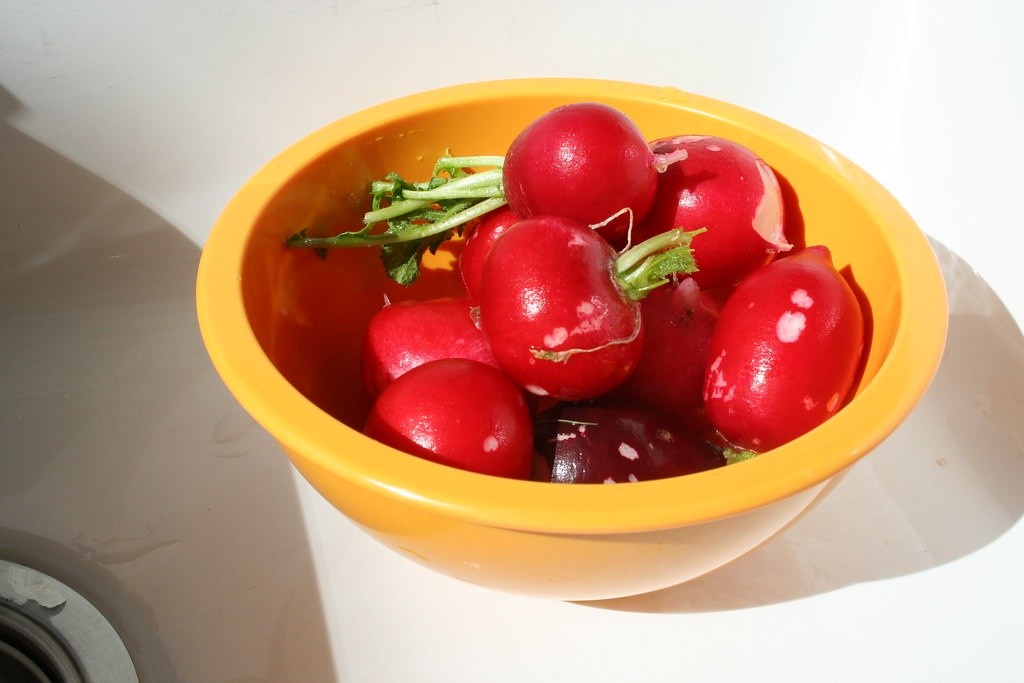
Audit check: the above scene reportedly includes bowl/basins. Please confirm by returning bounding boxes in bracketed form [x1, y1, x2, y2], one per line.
[196, 77, 949, 602]
